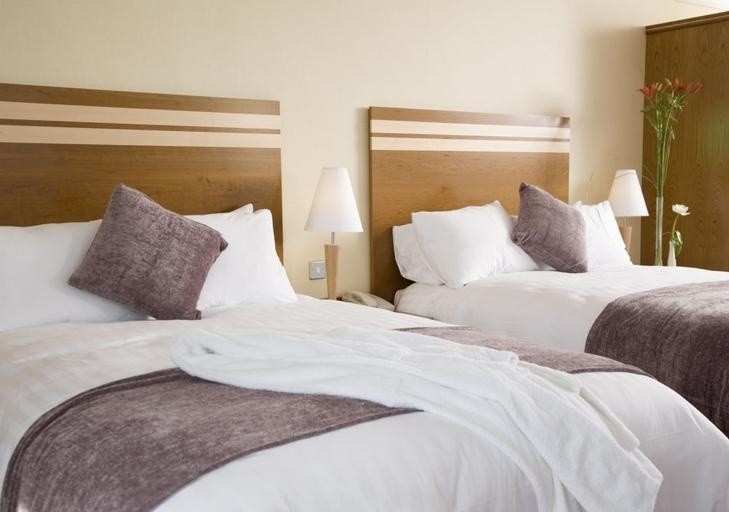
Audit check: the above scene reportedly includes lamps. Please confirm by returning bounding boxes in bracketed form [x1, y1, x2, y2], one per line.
[304, 167, 363, 300]
[608, 170, 650, 253]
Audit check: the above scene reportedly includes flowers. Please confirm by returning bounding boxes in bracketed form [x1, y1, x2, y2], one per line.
[661, 204, 692, 254]
[638, 77, 701, 264]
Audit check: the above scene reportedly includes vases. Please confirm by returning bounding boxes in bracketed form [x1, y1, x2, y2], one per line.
[667, 240, 678, 266]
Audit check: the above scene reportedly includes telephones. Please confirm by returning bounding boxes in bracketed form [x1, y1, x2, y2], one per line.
[342, 290, 395, 312]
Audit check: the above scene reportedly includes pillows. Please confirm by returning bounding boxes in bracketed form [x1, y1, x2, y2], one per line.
[391, 182, 632, 287]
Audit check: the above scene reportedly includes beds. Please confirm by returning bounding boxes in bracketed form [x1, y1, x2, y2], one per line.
[0, 83, 729, 511]
[370, 106, 728, 439]
